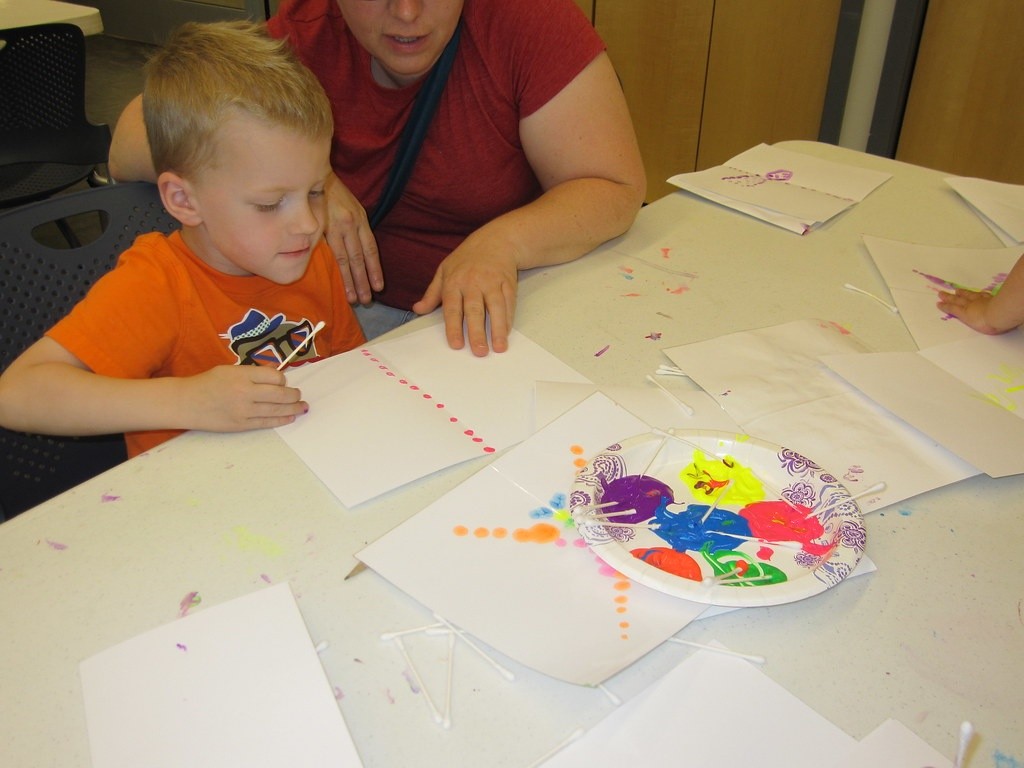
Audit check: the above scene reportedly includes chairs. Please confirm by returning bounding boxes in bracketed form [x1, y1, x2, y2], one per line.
[0, 22, 115, 249]
[0, 180, 183, 526]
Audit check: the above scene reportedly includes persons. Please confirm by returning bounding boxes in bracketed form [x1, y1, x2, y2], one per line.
[104, 1, 649, 355]
[936, 253, 1023, 334]
[1, 16, 368, 461]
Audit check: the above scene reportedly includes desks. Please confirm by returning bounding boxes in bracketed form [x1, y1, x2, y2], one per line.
[0, 0, 104, 50]
[0, 138, 1023, 765]
